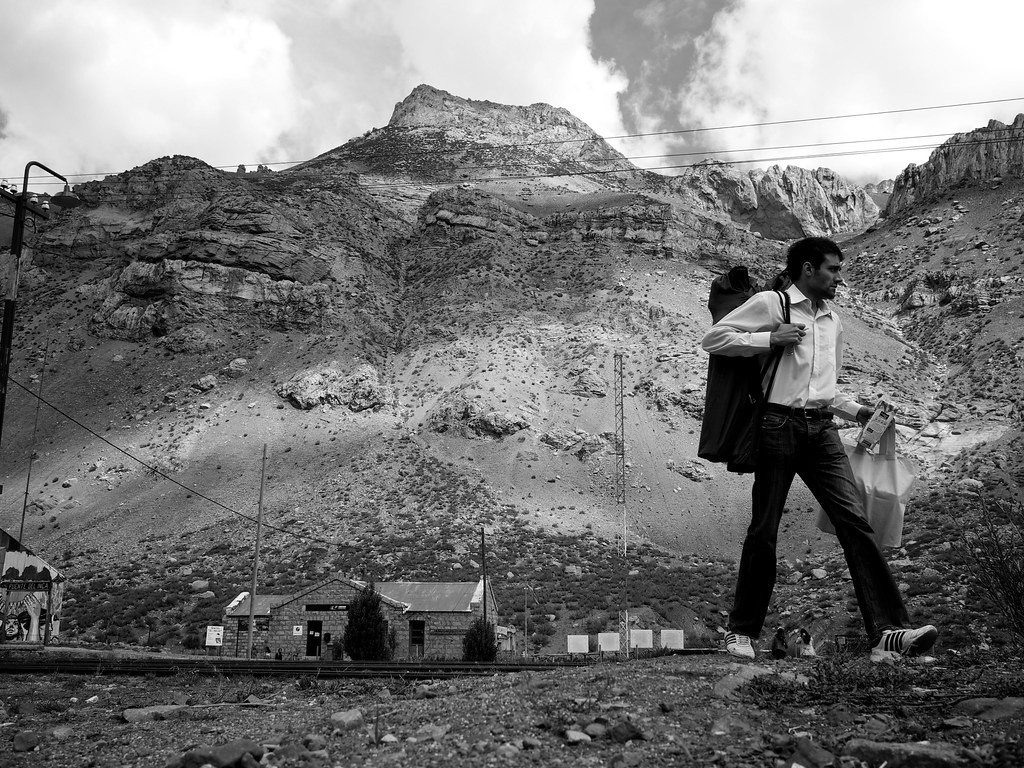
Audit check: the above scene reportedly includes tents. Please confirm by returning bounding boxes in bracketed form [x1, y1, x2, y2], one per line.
[0, 528, 66, 644]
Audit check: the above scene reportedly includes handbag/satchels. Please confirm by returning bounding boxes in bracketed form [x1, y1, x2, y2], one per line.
[814, 415, 919, 548]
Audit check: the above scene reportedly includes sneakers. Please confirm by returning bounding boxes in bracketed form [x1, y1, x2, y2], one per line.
[869, 624, 938, 663]
[724, 631, 757, 661]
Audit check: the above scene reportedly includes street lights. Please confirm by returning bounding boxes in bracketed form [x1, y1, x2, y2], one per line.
[0, 160, 82, 443]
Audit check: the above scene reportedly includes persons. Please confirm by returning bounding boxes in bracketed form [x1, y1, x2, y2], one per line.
[264, 647, 271, 658]
[702, 240, 938, 658]
[275, 648, 282, 660]
